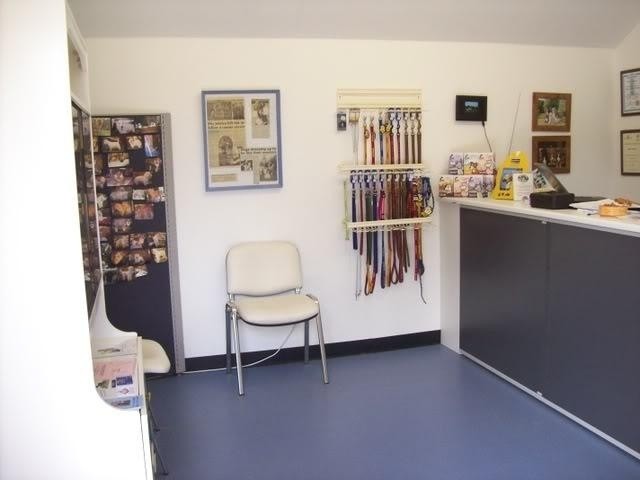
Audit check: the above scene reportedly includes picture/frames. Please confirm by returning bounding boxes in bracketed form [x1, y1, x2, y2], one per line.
[201, 88, 285, 191]
[619, 68, 640, 116]
[533, 136, 571, 173]
[619, 128, 640, 176]
[532, 92, 572, 131]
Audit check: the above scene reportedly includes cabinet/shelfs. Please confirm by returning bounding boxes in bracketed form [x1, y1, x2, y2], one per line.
[337, 88, 434, 241]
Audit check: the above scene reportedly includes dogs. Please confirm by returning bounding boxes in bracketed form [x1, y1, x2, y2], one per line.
[104, 139, 122, 152]
[133, 172, 152, 186]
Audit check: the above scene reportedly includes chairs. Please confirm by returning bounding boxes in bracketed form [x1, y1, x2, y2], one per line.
[223, 240, 330, 396]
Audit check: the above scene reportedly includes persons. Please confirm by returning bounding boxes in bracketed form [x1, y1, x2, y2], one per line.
[208, 99, 277, 180]
[538, 106, 563, 168]
